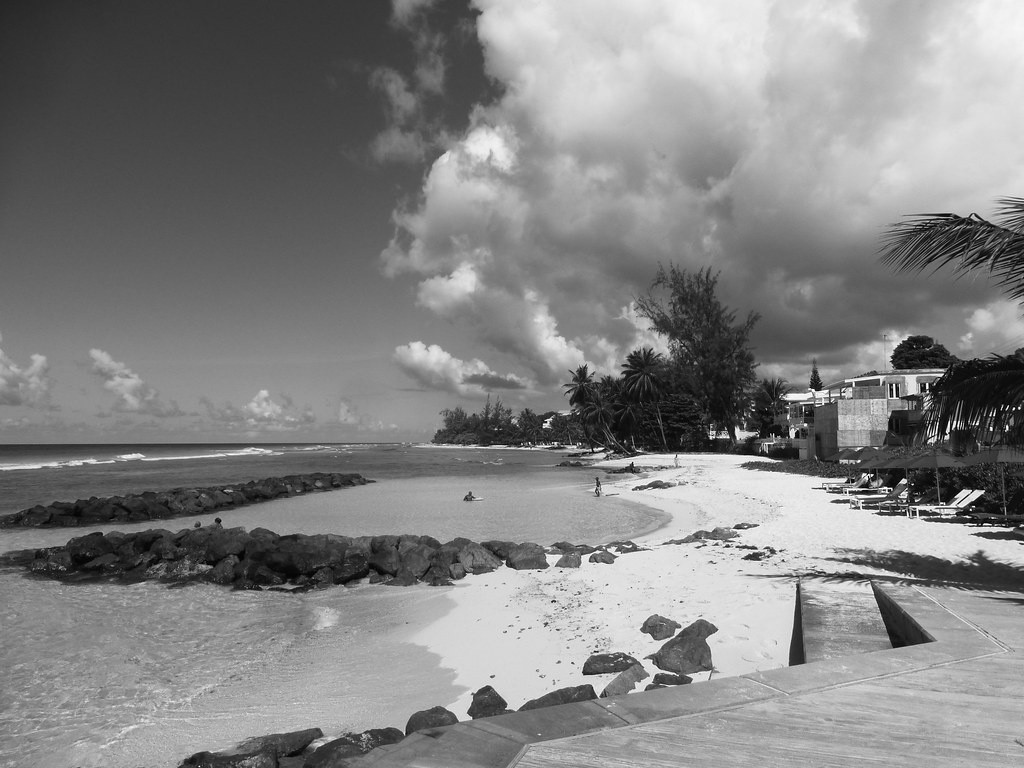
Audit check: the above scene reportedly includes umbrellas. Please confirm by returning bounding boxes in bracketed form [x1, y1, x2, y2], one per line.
[824, 445, 1024, 516]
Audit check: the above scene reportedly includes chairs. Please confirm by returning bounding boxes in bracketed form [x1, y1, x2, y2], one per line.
[819, 473, 985, 526]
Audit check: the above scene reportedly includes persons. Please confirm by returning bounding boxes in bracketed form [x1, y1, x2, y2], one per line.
[594, 477, 601, 496]
[463, 491, 475, 501]
